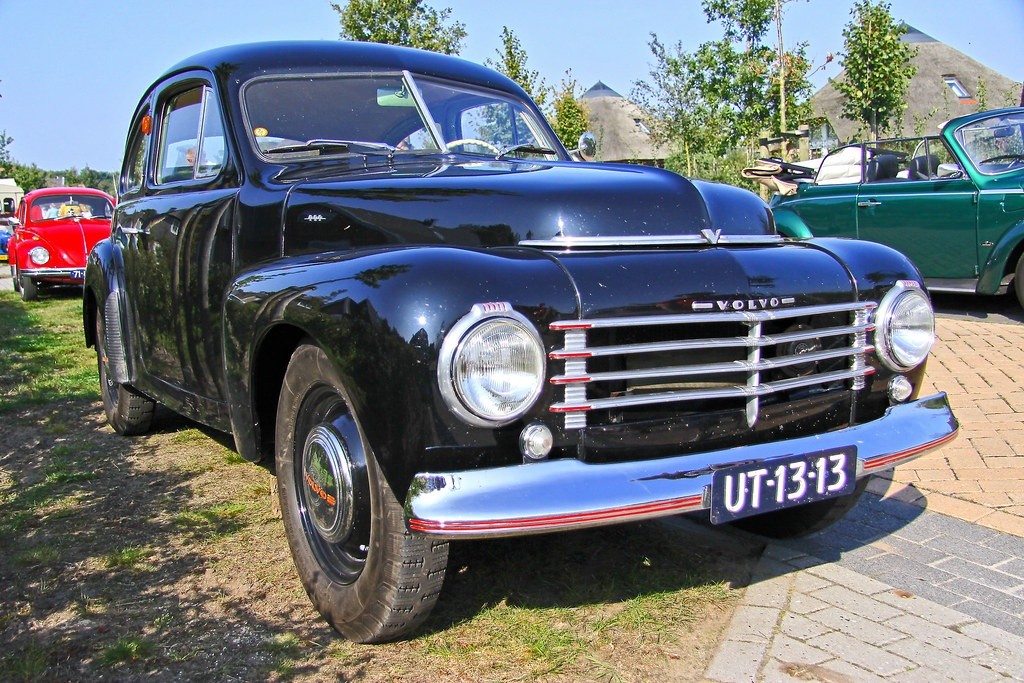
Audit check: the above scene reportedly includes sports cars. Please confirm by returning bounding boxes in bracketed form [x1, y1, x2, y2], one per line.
[768, 104, 1024, 313]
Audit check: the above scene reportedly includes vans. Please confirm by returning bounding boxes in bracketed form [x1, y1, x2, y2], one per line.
[0, 178, 27, 261]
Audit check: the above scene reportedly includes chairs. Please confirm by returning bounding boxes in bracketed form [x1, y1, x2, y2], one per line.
[908, 155, 940, 181]
[866, 155, 910, 184]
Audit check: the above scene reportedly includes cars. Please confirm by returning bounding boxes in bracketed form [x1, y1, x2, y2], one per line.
[82, 39, 961, 644]
[4, 186, 117, 302]
